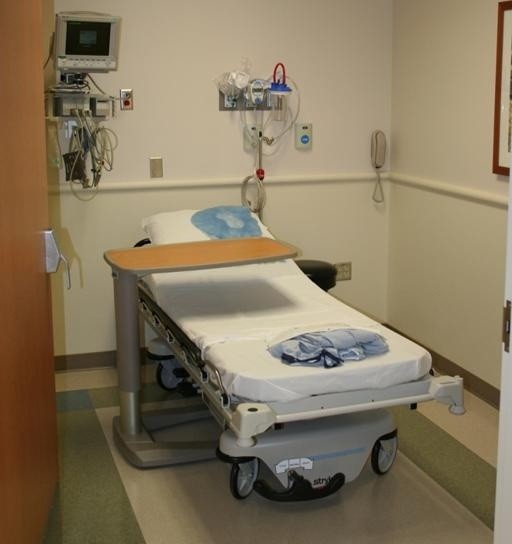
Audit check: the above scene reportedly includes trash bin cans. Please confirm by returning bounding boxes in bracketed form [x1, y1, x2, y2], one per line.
[295, 260, 339, 292]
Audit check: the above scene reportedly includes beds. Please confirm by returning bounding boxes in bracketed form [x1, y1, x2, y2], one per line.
[105, 206, 467, 508]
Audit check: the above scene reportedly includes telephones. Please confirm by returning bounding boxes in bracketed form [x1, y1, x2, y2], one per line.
[371, 131, 386, 168]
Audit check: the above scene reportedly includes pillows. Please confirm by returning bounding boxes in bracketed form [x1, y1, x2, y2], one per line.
[143, 207, 298, 282]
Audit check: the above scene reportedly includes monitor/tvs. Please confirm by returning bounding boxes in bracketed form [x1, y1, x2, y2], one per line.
[48, 10, 120, 74]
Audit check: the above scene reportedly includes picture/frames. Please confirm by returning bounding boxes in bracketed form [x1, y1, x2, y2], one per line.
[493, 1, 509, 175]
